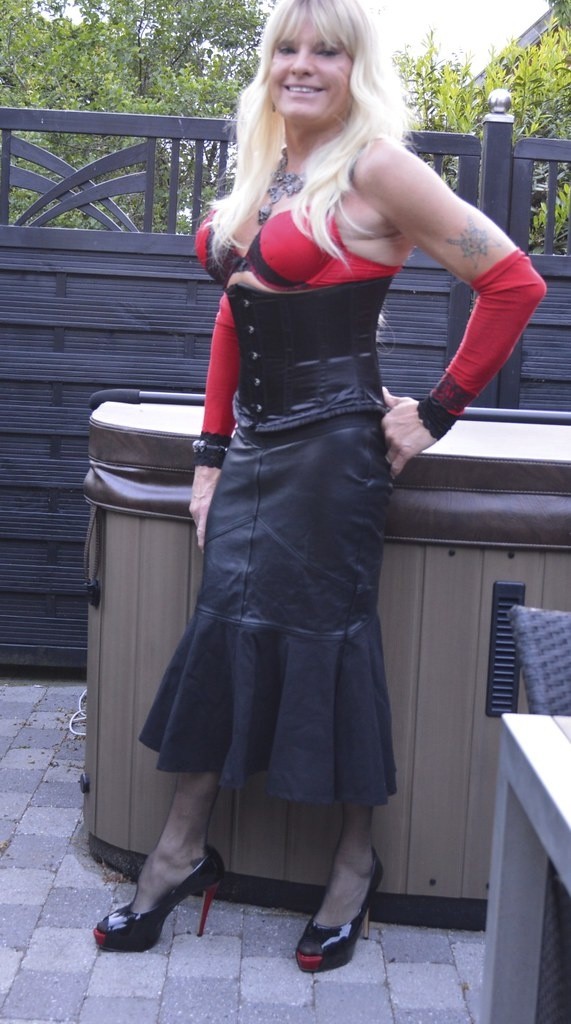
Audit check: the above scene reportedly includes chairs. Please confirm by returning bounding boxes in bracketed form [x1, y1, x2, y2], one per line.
[510, 604, 570, 1024]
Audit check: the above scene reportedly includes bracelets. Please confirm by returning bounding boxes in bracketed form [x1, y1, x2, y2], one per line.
[192, 440, 228, 453]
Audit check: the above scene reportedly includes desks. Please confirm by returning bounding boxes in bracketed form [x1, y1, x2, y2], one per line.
[481, 712, 570, 1024]
[80, 388, 570, 931]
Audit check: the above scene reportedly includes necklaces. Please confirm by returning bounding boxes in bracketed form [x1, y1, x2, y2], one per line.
[259, 146, 305, 226]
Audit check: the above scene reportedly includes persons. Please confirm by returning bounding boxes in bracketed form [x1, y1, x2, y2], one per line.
[93, 0, 547, 972]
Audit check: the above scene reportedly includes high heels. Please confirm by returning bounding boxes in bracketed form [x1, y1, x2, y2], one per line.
[295, 845, 383, 972]
[93, 844, 225, 953]
